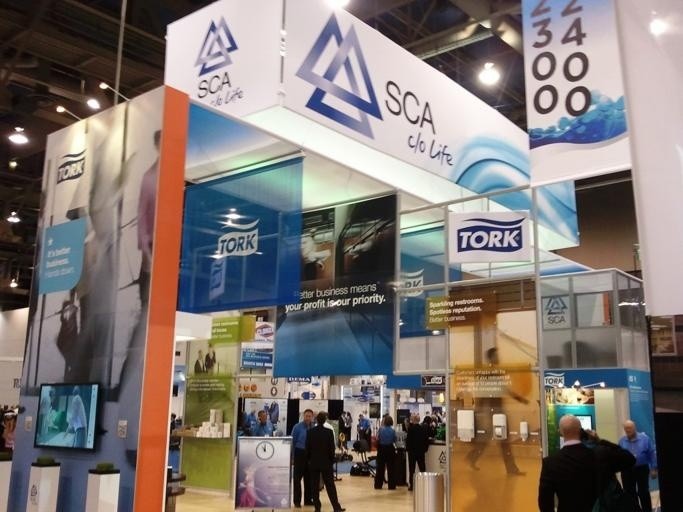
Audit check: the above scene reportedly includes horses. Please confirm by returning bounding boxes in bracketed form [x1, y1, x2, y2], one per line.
[580, 429, 590, 441]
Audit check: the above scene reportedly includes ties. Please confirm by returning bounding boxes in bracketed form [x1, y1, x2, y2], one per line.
[576, 415, 592, 430]
[34, 382, 100, 451]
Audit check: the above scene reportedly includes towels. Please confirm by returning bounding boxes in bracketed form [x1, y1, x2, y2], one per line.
[412, 471, 444, 512]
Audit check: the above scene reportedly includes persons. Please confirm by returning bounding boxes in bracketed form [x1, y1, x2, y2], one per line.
[252, 410, 274, 436]
[60, 384, 88, 448]
[462, 346, 528, 476]
[618, 419, 658, 512]
[538, 413, 636, 512]
[194, 344, 216, 373]
[137, 131, 160, 308]
[36, 385, 56, 437]
[291, 409, 446, 512]
